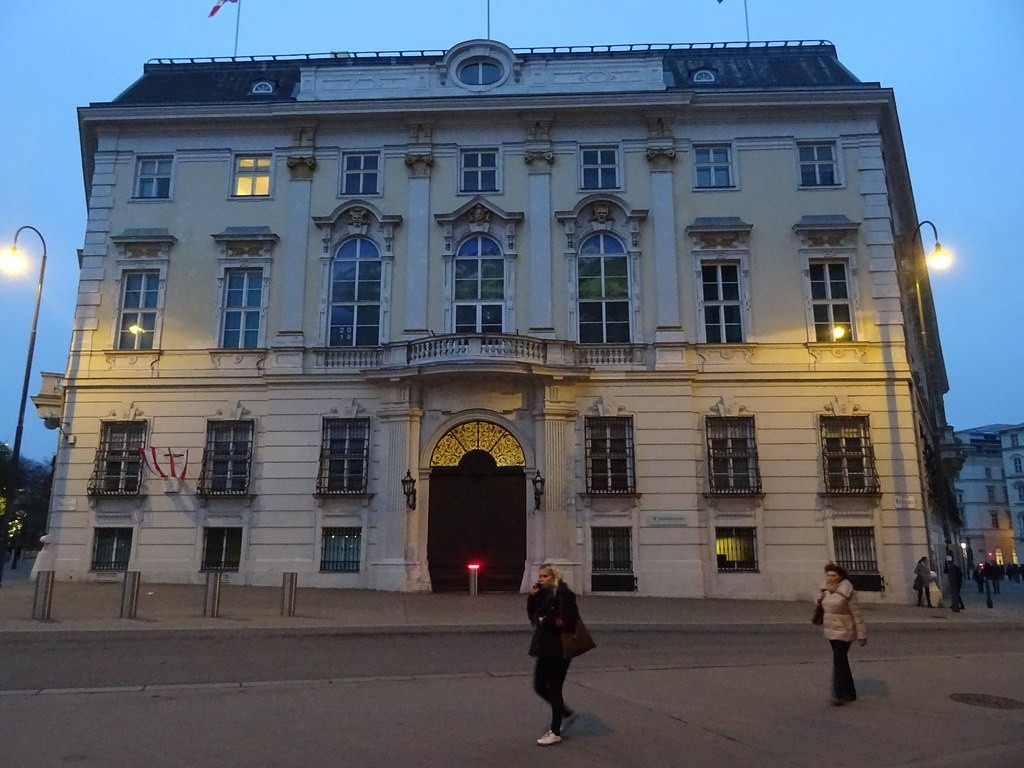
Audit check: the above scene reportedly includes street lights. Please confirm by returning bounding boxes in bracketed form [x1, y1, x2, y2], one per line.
[911, 220, 962, 611]
[1, 224, 49, 575]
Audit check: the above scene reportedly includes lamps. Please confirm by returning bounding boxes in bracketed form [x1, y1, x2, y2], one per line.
[533, 470, 545, 511]
[467, 565, 480, 595]
[401, 469, 416, 510]
[44, 416, 70, 440]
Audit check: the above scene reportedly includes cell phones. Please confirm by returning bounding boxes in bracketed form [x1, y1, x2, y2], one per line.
[534, 583, 540, 590]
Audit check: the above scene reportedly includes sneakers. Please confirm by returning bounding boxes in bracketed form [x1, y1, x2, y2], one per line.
[537, 729, 562, 745]
[560, 708, 579, 732]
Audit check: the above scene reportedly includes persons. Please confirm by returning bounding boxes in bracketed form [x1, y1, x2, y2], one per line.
[526, 563, 580, 745]
[914, 556, 936, 608]
[943, 556, 965, 613]
[972, 562, 1024, 594]
[820, 563, 868, 706]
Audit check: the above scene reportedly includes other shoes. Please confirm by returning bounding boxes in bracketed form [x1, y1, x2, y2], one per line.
[829, 695, 850, 706]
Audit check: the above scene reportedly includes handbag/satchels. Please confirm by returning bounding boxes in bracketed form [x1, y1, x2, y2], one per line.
[812, 604, 824, 625]
[559, 594, 596, 659]
[913, 574, 922, 591]
